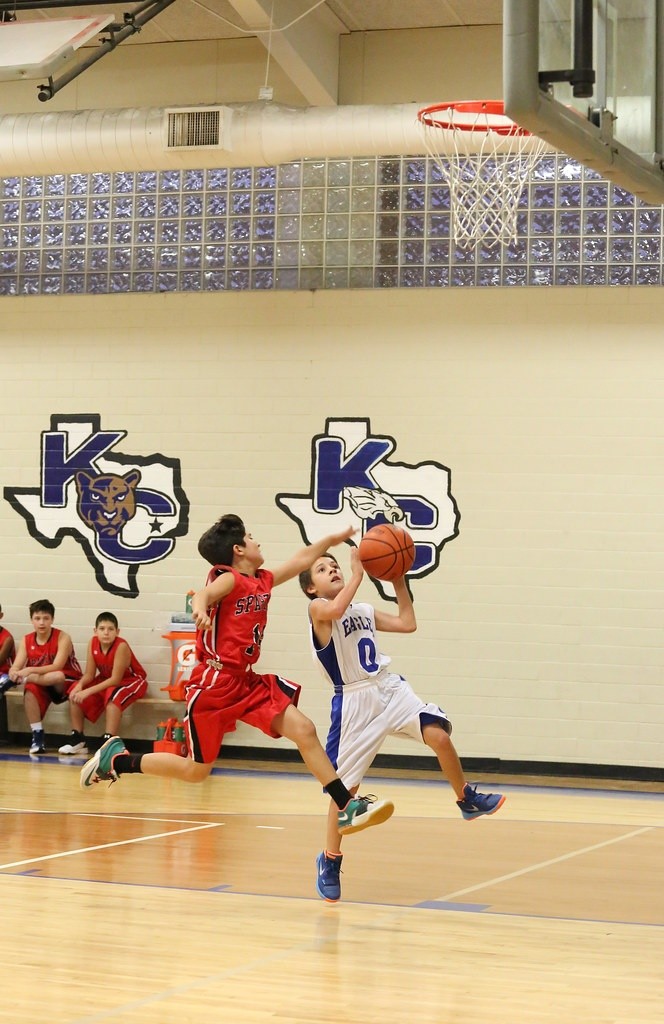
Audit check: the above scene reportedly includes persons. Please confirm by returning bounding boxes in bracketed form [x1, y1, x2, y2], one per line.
[79, 513, 394, 833]
[299, 547, 505, 902]
[8, 599, 82, 753]
[58, 612, 148, 754]
[0, 606, 16, 746]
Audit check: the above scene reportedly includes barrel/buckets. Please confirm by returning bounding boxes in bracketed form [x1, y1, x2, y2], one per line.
[160, 623, 198, 702]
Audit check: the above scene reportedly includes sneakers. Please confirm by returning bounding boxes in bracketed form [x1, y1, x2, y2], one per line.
[337, 793, 395, 835]
[456, 782, 506, 821]
[316, 851, 344, 902]
[30, 727, 47, 754]
[0, 674, 22, 700]
[58, 729, 89, 755]
[97, 733, 112, 750]
[80, 735, 130, 791]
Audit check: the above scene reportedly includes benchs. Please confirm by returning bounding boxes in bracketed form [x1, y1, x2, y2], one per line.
[3, 686, 191, 703]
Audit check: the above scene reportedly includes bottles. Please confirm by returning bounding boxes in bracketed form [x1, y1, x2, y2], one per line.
[186, 590, 195, 612]
[182, 723, 186, 741]
[167, 718, 177, 740]
[172, 722, 183, 741]
[157, 721, 167, 740]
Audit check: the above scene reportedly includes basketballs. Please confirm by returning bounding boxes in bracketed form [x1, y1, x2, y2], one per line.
[360, 523, 417, 581]
